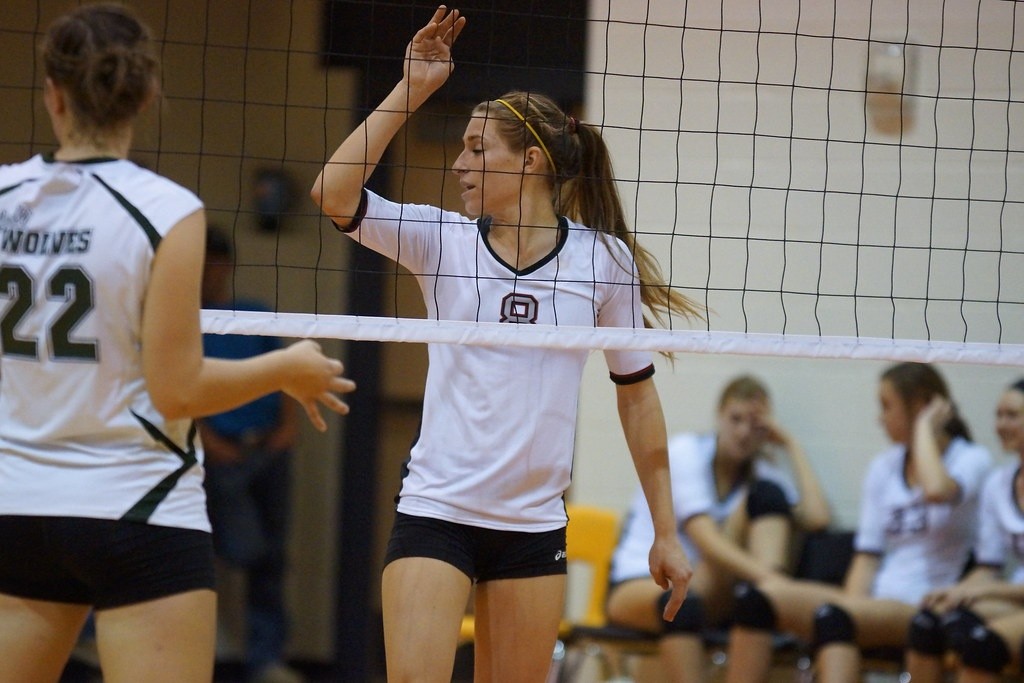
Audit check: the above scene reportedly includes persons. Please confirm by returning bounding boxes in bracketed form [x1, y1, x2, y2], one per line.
[603, 362, 1024, 683]
[0, 2, 356, 683]
[308, 4, 694, 683]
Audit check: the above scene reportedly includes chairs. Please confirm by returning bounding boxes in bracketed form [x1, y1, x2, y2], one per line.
[454, 503, 856, 683]
